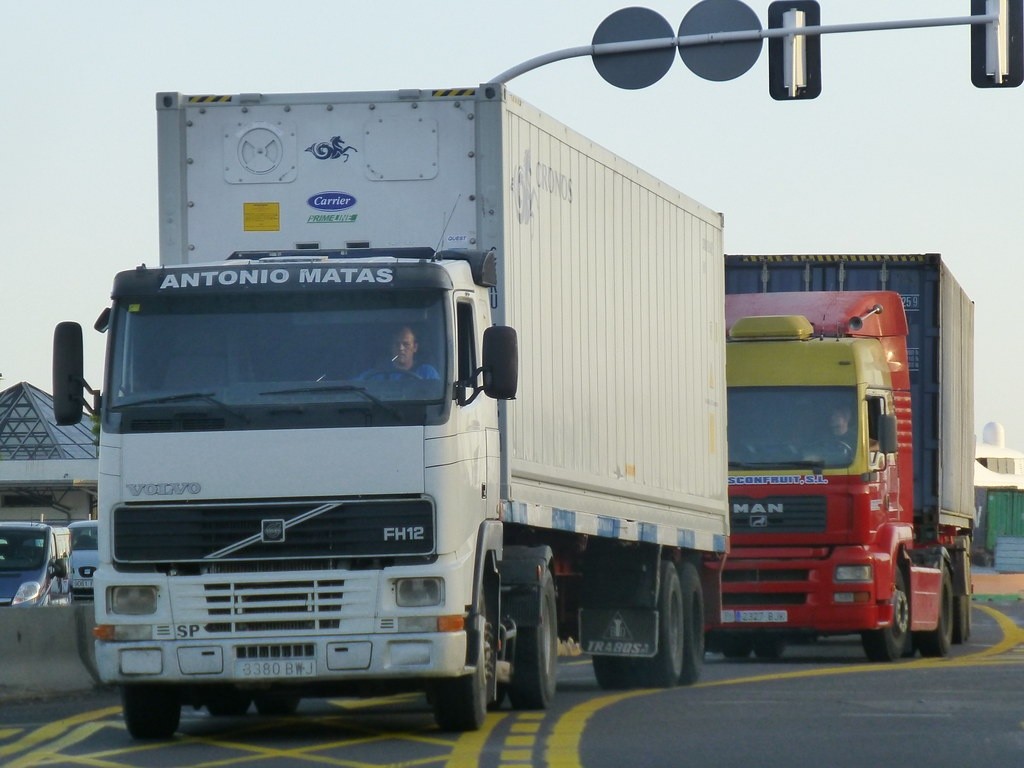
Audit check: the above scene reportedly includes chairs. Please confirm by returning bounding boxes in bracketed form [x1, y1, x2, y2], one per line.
[161, 325, 255, 390]
[77, 535, 93, 548]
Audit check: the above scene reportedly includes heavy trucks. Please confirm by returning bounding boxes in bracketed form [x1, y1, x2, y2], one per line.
[700, 251, 981, 662]
[49, 82, 728, 739]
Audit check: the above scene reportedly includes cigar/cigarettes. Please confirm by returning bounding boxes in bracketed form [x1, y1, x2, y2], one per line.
[391, 355, 398, 362]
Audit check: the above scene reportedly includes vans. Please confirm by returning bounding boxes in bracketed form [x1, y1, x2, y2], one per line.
[0, 519, 74, 608]
[65, 518, 98, 594]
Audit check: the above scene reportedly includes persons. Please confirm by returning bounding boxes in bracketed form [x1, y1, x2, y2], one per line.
[804, 409, 879, 454]
[358, 323, 440, 382]
